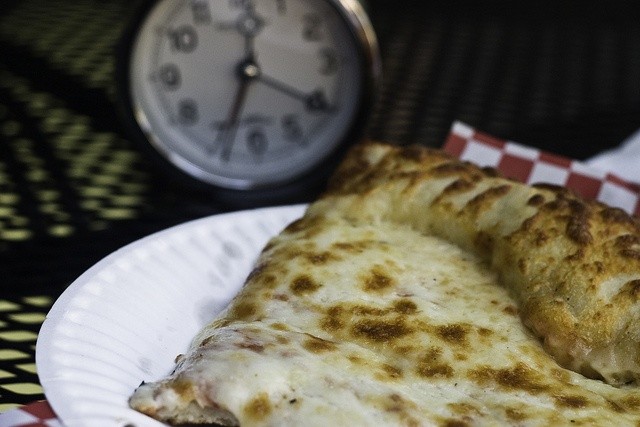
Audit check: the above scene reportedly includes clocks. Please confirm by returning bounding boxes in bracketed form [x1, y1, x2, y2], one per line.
[116, 1, 382, 207]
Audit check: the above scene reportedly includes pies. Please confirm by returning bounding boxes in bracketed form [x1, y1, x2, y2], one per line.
[127, 141, 640, 427]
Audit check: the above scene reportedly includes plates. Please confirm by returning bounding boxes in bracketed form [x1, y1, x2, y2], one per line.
[35, 203, 312, 427]
